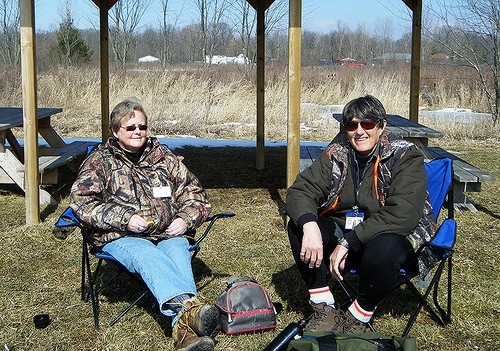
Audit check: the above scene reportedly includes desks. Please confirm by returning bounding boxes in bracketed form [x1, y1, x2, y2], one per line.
[333, 114, 443, 159]
[0, 107, 67, 205]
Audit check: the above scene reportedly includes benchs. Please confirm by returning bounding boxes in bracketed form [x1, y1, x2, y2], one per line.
[298, 146, 323, 173]
[19, 141, 91, 185]
[428, 147, 496, 192]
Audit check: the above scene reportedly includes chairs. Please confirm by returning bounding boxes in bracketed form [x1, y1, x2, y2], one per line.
[324, 157, 458, 338]
[54, 144, 236, 329]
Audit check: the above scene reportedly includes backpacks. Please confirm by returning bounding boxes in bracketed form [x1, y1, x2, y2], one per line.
[214, 275, 278, 335]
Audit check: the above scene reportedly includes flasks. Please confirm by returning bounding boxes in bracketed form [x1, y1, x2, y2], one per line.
[263, 317, 310, 351]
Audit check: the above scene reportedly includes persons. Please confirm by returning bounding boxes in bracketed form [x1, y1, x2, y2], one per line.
[70, 100, 221, 351]
[284, 94, 435, 333]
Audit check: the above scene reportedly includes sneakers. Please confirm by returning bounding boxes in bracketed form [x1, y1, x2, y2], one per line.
[171, 319, 215, 351]
[332, 310, 367, 336]
[178, 296, 220, 337]
[303, 299, 340, 337]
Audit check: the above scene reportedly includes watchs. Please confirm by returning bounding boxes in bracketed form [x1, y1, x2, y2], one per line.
[337, 237, 350, 249]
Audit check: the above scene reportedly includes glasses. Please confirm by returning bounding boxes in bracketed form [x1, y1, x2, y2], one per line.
[344, 120, 380, 131]
[120, 125, 148, 131]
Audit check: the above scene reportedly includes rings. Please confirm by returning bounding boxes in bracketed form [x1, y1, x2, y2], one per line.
[137, 225, 141, 231]
